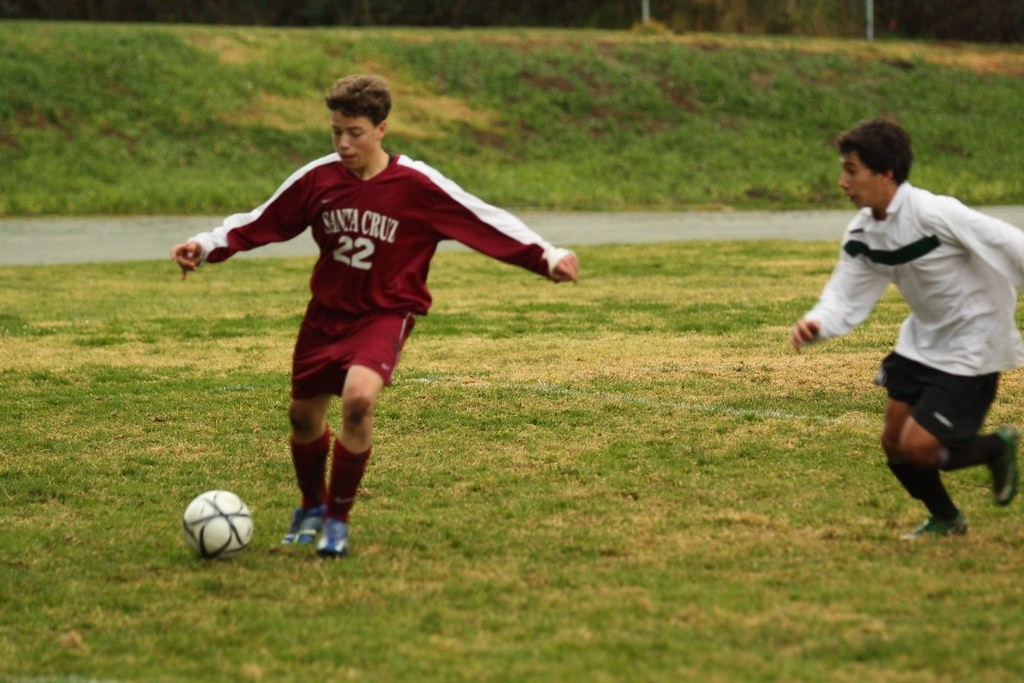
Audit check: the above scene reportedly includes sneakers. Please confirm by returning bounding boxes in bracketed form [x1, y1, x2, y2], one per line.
[994, 425, 1020, 505]
[318, 519, 348, 555]
[282, 505, 326, 547]
[900, 510, 968, 543]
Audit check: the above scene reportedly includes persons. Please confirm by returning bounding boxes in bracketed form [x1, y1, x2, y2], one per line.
[787, 114, 1024, 535]
[169, 72, 579, 560]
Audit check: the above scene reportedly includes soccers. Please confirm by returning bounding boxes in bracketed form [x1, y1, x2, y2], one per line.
[181, 488, 254, 562]
